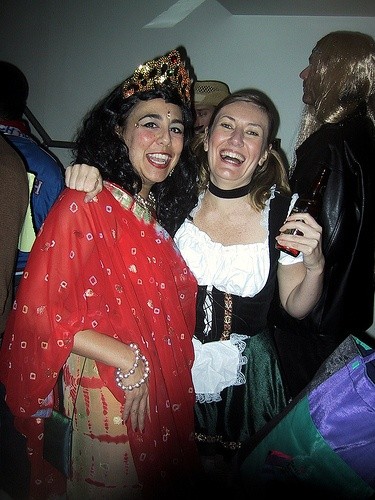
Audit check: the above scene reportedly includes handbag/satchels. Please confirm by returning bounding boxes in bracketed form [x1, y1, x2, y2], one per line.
[237, 334, 375, 500]
[43, 409, 73, 478]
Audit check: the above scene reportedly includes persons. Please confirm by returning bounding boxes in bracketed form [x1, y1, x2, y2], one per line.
[1, 64, 64, 300]
[191, 82, 230, 133]
[289, 32, 375, 362]
[65, 89, 325, 493]
[1, 75, 198, 496]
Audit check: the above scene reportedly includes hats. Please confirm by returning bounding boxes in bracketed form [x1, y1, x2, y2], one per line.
[192, 81, 231, 107]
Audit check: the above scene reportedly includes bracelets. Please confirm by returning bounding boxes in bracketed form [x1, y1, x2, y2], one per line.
[115, 344, 151, 392]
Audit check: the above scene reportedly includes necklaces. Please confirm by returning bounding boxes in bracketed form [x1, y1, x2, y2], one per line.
[134, 191, 157, 211]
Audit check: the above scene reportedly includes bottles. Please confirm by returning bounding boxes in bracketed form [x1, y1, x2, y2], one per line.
[275, 168, 332, 256]
